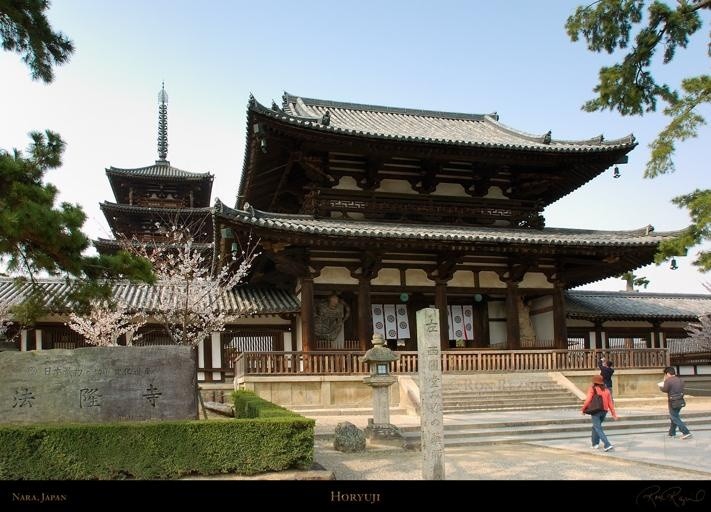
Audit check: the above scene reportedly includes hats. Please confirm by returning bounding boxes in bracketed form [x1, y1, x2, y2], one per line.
[591, 375, 604, 385]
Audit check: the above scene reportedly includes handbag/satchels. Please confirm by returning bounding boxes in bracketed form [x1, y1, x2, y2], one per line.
[668, 394, 686, 410]
[583, 394, 604, 415]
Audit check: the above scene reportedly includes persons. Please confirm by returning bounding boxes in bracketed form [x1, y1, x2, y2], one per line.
[582, 356, 618, 453]
[657, 366, 694, 439]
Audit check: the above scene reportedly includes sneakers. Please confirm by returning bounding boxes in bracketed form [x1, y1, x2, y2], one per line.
[604, 445, 613, 452]
[681, 433, 694, 439]
[590, 444, 600, 450]
